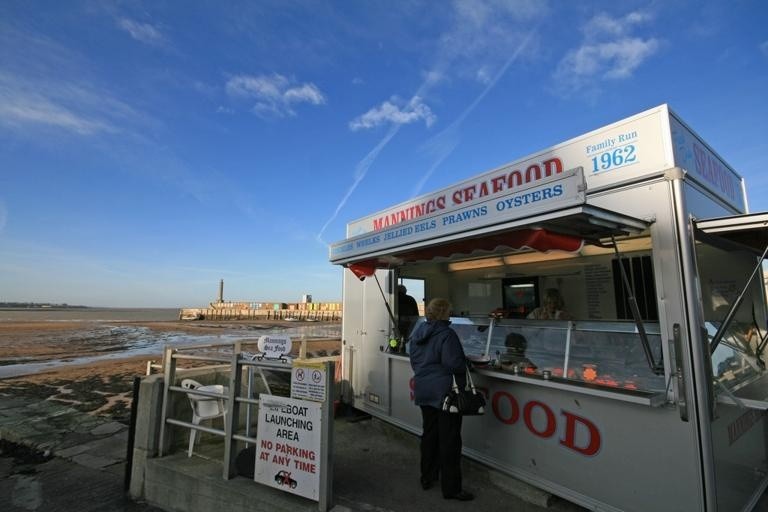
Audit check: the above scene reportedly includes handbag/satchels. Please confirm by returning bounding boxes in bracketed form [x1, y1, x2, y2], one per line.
[441, 365, 486, 417]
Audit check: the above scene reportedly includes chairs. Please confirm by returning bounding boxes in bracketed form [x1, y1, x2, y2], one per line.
[181, 378, 228, 458]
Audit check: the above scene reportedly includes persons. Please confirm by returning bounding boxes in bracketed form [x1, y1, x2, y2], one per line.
[526, 287, 575, 355]
[488, 331, 536, 374]
[394, 284, 420, 354]
[409, 298, 475, 502]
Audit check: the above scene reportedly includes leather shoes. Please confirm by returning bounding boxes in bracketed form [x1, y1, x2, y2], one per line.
[443, 490, 474, 501]
[420, 475, 432, 491]
